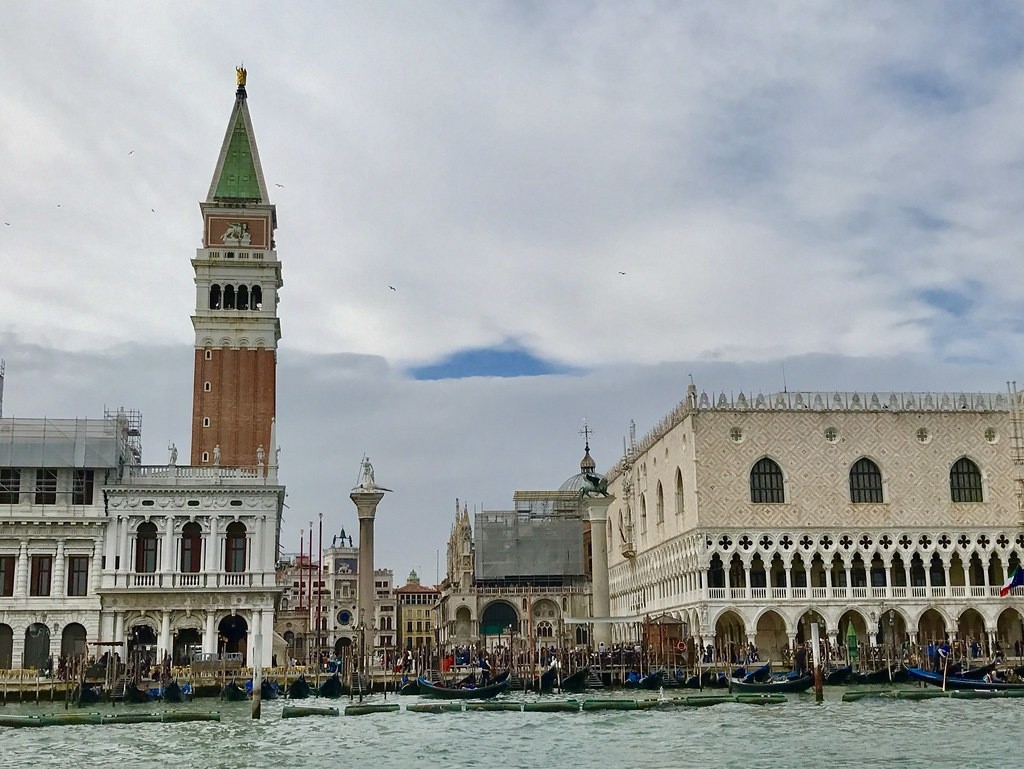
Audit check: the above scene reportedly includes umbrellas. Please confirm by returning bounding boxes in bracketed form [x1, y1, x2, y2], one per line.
[370, 470, 375, 483]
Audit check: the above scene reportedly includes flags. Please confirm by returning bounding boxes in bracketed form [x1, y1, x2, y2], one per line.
[1000, 564, 1024, 597]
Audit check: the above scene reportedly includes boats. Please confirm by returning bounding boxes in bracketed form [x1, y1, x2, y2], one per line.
[288, 672, 312, 699]
[224, 681, 250, 701]
[163, 678, 183, 702]
[401, 659, 1024, 700]
[261, 677, 278, 699]
[319, 665, 342, 699]
[127, 681, 152, 703]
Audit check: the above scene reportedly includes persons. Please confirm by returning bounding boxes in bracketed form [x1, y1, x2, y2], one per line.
[256, 444, 265, 466]
[168, 443, 178, 464]
[47, 630, 1024, 700]
[117, 406, 126, 426]
[213, 444, 221, 465]
[360, 456, 373, 483]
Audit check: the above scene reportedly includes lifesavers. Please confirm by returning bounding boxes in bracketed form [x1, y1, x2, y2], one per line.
[677, 642, 686, 651]
